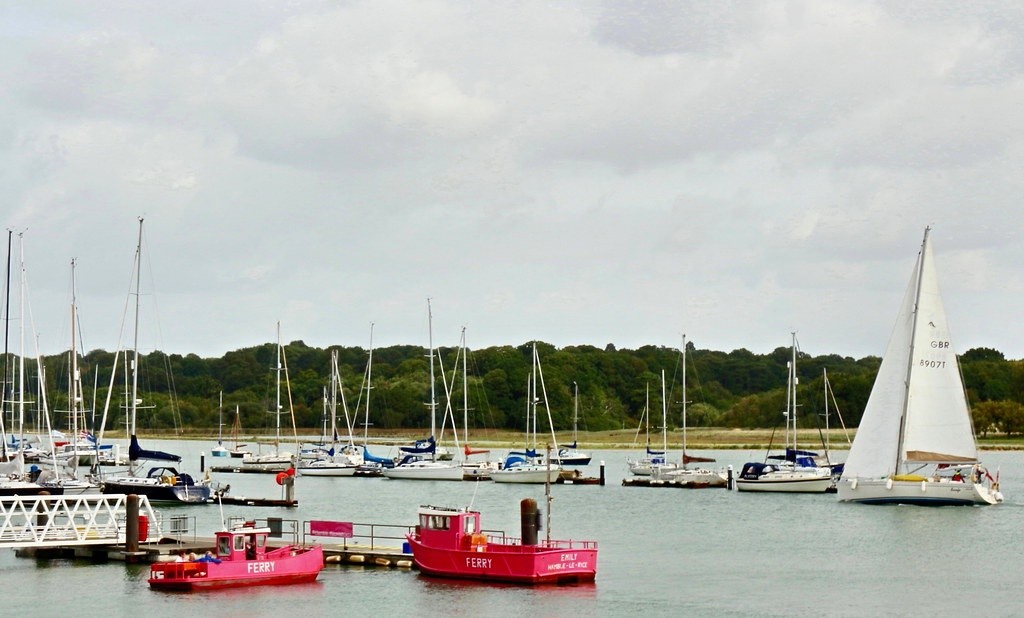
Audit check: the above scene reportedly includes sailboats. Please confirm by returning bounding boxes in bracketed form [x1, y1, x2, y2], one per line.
[834, 225, 1005, 505]
[0, 217, 844, 507]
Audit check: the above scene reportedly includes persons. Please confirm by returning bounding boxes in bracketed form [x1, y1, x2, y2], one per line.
[950, 467, 965, 484]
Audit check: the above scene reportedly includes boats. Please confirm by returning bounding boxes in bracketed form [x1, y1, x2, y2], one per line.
[146, 529, 325, 586]
[404, 510, 599, 584]
